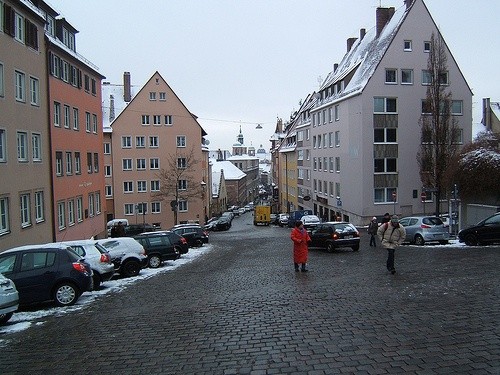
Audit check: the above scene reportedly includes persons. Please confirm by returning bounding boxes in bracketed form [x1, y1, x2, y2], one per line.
[377, 215, 406, 275]
[290, 221, 312, 272]
[115, 221, 126, 238]
[367, 212, 390, 247]
[322, 212, 341, 221]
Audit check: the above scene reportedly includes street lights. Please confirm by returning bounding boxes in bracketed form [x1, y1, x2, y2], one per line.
[392, 190, 397, 214]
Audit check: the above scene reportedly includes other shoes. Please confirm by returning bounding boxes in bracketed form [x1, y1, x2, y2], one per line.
[391, 270, 396, 275]
[302, 269, 308, 272]
[370, 244, 373, 246]
[387, 266, 391, 271]
[374, 245, 376, 247]
[295, 268, 299, 272]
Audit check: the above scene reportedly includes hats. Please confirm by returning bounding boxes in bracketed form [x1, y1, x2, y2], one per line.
[391, 215, 398, 222]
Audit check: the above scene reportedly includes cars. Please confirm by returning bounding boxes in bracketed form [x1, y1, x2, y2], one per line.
[98, 237, 149, 278]
[46, 240, 115, 292]
[299, 215, 320, 230]
[0, 272, 19, 324]
[0, 243, 94, 307]
[308, 222, 361, 253]
[133, 234, 177, 269]
[140, 231, 189, 259]
[169, 183, 312, 248]
[399, 216, 450, 245]
[457, 211, 500, 246]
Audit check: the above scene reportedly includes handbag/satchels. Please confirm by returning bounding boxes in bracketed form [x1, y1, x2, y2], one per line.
[368, 229, 371, 235]
